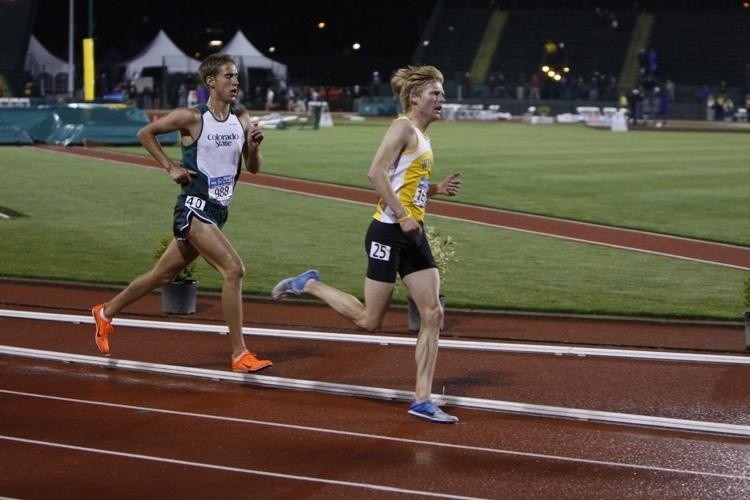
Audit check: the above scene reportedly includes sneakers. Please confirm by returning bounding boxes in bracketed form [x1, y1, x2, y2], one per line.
[272, 270, 321, 300]
[231, 350, 273, 373]
[92, 303, 113, 354]
[408, 395, 459, 422]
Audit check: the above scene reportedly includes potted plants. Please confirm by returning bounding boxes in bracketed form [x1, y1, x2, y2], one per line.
[152, 231, 199, 315]
[394, 223, 456, 332]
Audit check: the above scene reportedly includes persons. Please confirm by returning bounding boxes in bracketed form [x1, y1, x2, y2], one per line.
[92, 56, 272, 374]
[272, 65, 460, 425]
[455, 71, 750, 129]
[121, 71, 382, 113]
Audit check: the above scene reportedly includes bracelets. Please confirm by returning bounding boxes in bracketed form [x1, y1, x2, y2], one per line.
[397, 207, 412, 223]
